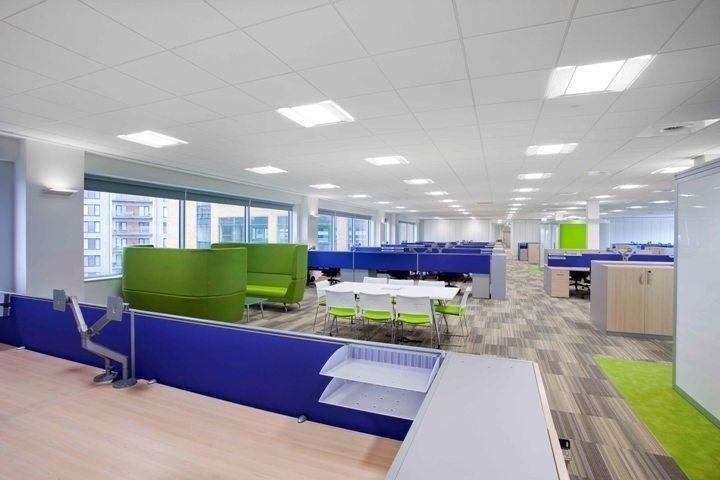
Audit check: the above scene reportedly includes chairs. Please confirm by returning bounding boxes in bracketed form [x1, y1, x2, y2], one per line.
[375, 269, 465, 293]
[308, 271, 315, 287]
[313, 276, 473, 349]
[321, 267, 342, 284]
[570, 271, 590, 299]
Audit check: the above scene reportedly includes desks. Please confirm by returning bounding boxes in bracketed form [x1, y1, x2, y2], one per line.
[1, 342, 405, 478]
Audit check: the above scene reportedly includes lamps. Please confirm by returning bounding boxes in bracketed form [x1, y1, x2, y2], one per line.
[41, 187, 74, 196]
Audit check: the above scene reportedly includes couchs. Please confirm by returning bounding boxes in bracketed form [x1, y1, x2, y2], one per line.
[211, 242, 308, 312]
[123, 244, 249, 323]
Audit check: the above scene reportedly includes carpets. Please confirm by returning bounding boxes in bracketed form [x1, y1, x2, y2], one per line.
[594, 356, 720, 480]
